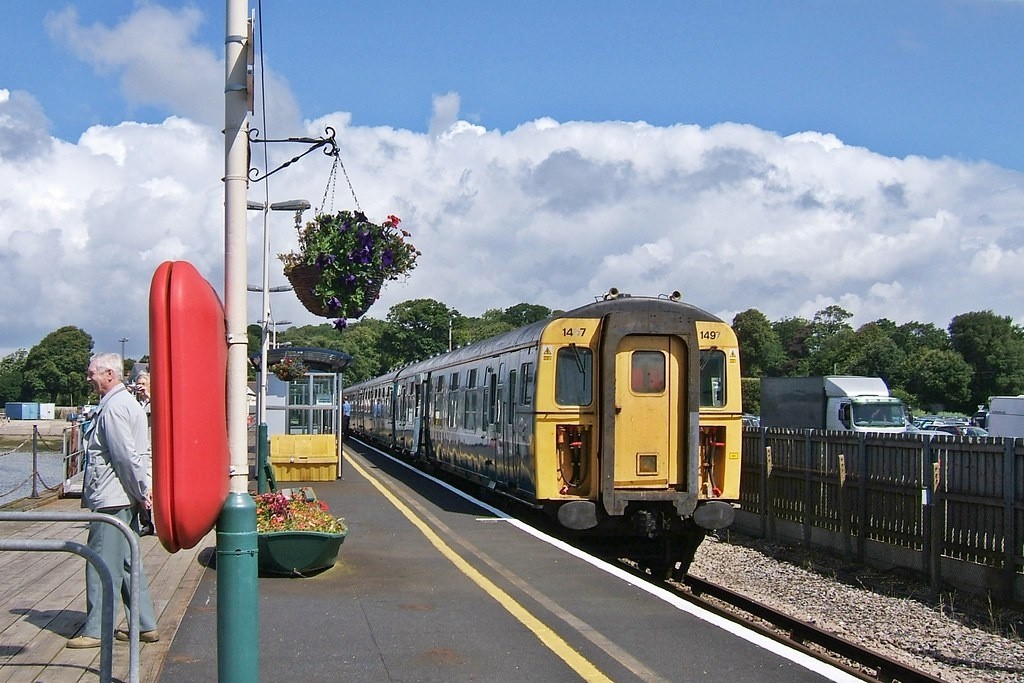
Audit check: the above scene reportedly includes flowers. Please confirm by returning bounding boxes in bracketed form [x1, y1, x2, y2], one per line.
[276, 206, 422, 333]
[268, 358, 310, 378]
[253, 493, 346, 533]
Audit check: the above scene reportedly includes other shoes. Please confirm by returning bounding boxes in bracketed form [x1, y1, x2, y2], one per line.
[67, 635, 101, 648]
[139, 522, 154, 537]
[114, 628, 159, 642]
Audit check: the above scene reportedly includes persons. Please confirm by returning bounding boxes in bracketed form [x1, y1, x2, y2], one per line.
[342, 396, 350, 441]
[67, 352, 159, 648]
[870, 405, 887, 420]
[320, 409, 328, 434]
[970, 417, 980, 428]
[122, 369, 155, 537]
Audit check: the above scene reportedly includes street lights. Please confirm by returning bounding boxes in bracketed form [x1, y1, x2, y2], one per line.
[257, 320, 293, 351]
[117, 338, 129, 376]
[245, 197, 312, 482]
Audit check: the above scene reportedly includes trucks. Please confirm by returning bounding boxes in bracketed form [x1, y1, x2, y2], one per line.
[759, 374, 913, 434]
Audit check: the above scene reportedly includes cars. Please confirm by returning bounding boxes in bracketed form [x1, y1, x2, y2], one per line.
[905, 414, 990, 444]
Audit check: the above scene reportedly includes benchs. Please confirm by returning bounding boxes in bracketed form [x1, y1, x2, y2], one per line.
[264, 462, 316, 502]
[268, 434, 338, 481]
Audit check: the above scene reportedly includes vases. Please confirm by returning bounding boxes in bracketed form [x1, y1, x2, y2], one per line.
[258, 528, 349, 579]
[278, 374, 298, 382]
[281, 262, 384, 319]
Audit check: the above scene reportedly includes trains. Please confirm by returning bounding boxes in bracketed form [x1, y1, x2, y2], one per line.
[341, 287, 745, 582]
